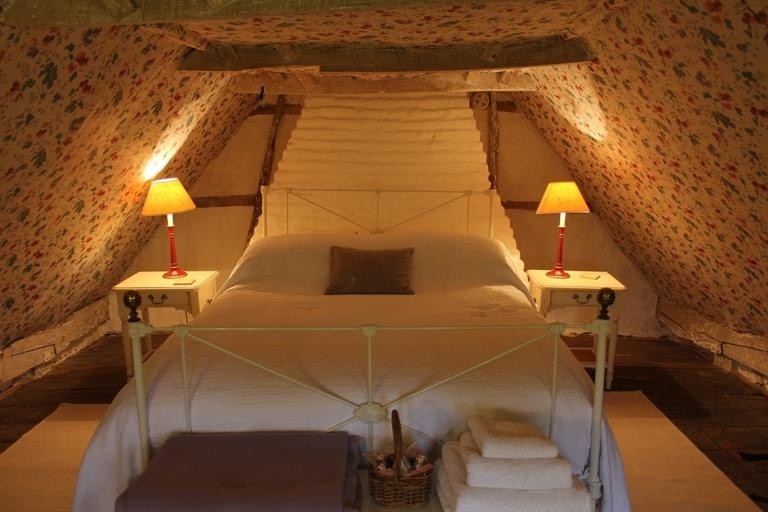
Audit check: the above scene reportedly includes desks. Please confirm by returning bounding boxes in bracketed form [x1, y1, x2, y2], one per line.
[111, 269, 220, 383]
[526, 268, 629, 391]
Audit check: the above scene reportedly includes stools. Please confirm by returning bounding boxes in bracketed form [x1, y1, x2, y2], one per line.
[113, 429, 361, 512]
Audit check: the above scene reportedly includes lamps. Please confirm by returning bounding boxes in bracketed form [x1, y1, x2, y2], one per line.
[140, 177, 197, 280]
[535, 179, 591, 279]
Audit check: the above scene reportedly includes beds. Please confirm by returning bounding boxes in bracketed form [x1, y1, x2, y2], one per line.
[69, 183, 635, 512]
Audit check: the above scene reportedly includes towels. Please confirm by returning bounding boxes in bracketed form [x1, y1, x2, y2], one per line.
[432, 416, 590, 512]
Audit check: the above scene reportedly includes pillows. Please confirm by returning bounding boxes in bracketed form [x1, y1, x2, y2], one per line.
[395, 229, 538, 311]
[322, 245, 416, 296]
[217, 229, 374, 295]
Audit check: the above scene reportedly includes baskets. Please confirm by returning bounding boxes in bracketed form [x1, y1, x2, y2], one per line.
[370, 409, 433, 510]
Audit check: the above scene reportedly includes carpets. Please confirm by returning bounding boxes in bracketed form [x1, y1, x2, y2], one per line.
[1, 390, 763, 511]
